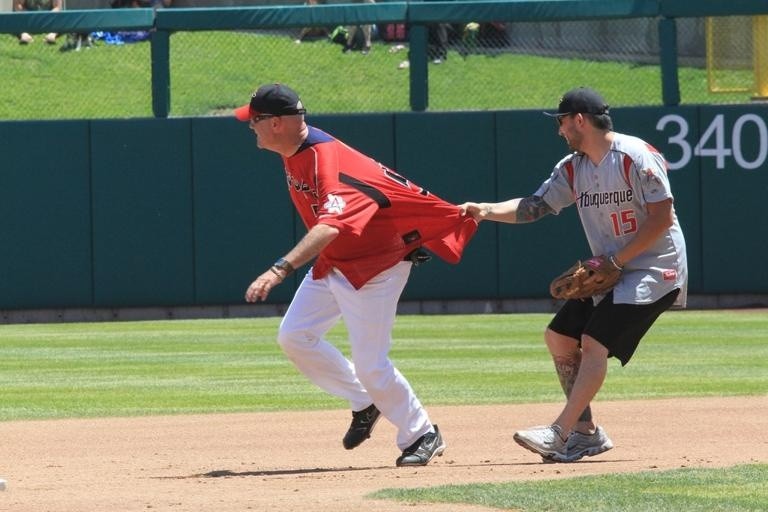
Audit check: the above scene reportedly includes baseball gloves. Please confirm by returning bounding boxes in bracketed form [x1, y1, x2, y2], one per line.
[550, 251, 623, 299]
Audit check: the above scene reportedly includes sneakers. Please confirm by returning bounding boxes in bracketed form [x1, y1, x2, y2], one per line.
[553, 425, 616, 464]
[339, 401, 383, 450]
[394, 423, 449, 470]
[511, 424, 571, 462]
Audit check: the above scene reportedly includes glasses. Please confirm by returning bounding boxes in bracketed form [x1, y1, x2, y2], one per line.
[555, 115, 575, 126]
[251, 114, 276, 123]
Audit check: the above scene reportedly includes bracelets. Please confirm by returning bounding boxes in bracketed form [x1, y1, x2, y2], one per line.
[274, 257, 294, 276]
[269, 265, 285, 281]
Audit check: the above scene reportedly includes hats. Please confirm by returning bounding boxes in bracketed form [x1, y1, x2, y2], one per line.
[231, 81, 307, 123]
[540, 87, 610, 120]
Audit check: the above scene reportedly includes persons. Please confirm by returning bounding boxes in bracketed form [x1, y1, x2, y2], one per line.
[13, 1, 65, 43]
[455, 84, 693, 463]
[395, 22, 452, 71]
[292, 0, 334, 47]
[610, 255, 623, 272]
[232, 80, 480, 466]
[342, 0, 376, 56]
